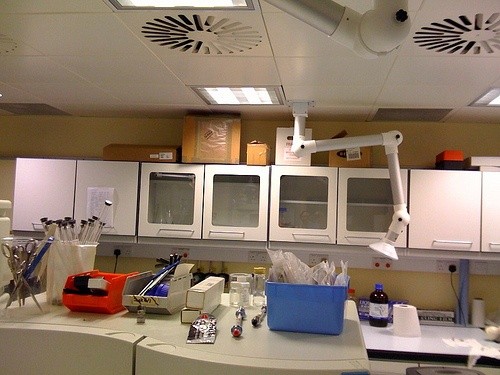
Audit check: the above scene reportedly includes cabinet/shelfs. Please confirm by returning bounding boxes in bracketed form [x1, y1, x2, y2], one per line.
[11, 157, 139, 235]
[138, 163, 270, 242]
[269, 165, 408, 249]
[408, 168, 500, 253]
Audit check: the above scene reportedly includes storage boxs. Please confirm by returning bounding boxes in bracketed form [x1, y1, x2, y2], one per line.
[247, 140, 272, 165]
[264, 274, 350, 335]
[329, 146, 372, 168]
[274, 127, 312, 166]
[181, 276, 225, 325]
[435, 150, 500, 171]
[103, 144, 181, 162]
[358, 297, 408, 323]
[182, 113, 242, 164]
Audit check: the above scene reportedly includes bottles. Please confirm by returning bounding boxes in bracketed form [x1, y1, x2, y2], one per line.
[369, 283, 389, 327]
[346, 288, 357, 302]
[252, 266, 267, 307]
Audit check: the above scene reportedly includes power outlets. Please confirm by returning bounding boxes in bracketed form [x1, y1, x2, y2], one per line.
[436, 260, 460, 274]
[308, 253, 329, 267]
[112, 244, 132, 256]
[248, 251, 272, 264]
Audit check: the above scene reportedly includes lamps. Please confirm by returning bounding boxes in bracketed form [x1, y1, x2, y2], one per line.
[291, 102, 410, 260]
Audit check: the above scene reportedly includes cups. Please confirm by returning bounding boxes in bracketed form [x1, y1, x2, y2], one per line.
[229, 273, 253, 308]
[46, 241, 98, 306]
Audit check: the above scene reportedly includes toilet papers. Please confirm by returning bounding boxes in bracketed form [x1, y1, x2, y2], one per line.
[470, 299, 486, 327]
[391, 303, 422, 338]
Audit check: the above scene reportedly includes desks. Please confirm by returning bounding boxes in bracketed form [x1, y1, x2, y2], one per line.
[361, 320, 500, 375]
[0, 289, 371, 375]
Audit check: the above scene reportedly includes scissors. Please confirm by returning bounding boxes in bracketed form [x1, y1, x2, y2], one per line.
[2, 240, 43, 313]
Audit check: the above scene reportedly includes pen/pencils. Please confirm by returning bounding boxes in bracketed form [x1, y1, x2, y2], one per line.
[170, 255, 173, 267]
[174, 254, 178, 264]
[178, 255, 183, 262]
